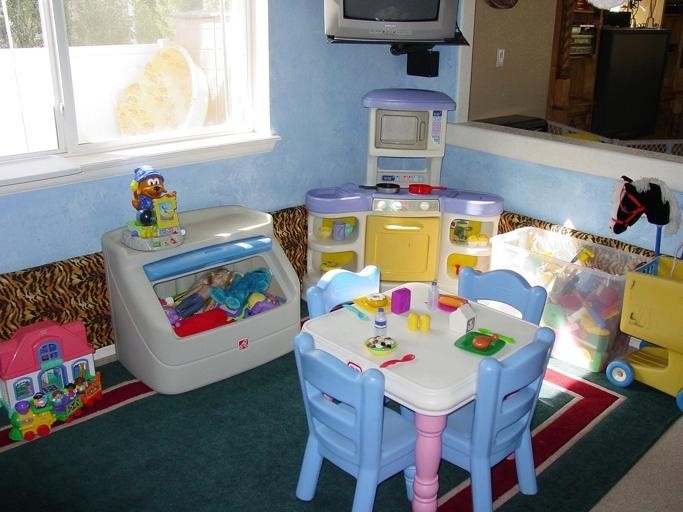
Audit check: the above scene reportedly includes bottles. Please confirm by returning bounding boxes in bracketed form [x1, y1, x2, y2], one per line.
[427, 281, 439, 312]
[374, 305, 387, 337]
[406, 313, 431, 332]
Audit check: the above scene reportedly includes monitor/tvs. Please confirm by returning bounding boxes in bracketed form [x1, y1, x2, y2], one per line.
[324, 0, 458, 44]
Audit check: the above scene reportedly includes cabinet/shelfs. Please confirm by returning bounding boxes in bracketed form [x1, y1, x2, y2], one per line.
[544, 0, 603, 134]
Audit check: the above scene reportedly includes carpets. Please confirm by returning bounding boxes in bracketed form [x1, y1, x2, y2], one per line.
[0, 295, 683, 510]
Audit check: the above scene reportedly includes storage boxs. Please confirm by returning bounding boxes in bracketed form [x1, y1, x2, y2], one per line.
[487, 226, 652, 375]
[102, 205, 300, 395]
